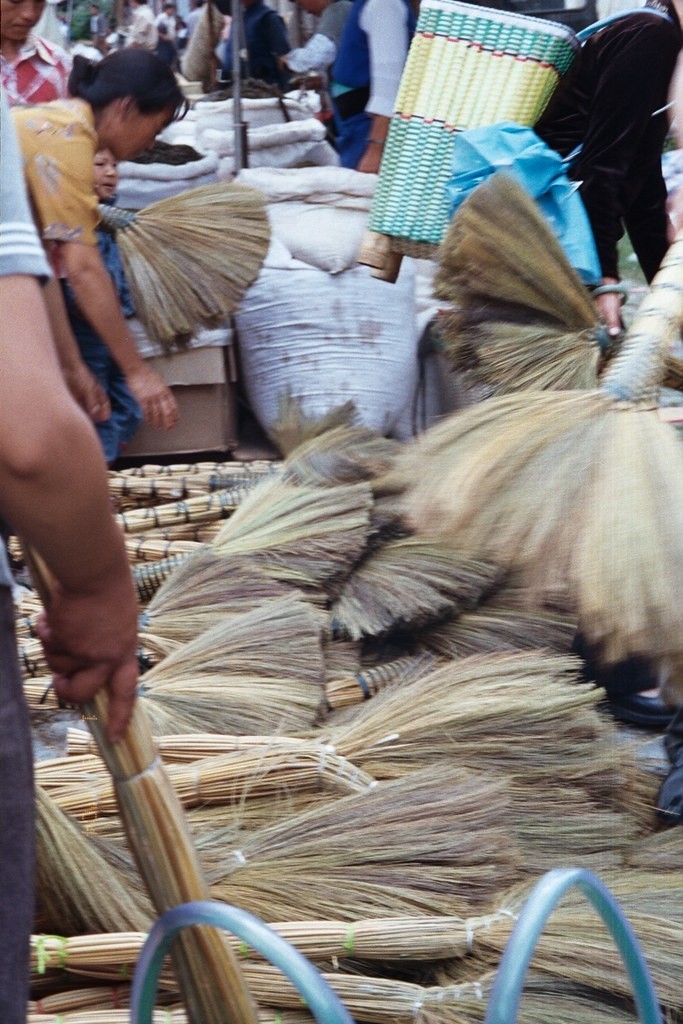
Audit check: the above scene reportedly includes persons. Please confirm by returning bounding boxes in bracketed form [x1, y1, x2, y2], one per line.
[187, 0, 204, 39]
[18, 53, 197, 429]
[0, 87, 141, 1022]
[156, 4, 179, 52]
[62, 149, 143, 468]
[1, 0, 70, 102]
[90, 5, 107, 56]
[538, 0, 683, 335]
[124, 0, 157, 51]
[280, 0, 353, 74]
[331, 0, 412, 174]
[220, 0, 292, 81]
[100, 25, 118, 50]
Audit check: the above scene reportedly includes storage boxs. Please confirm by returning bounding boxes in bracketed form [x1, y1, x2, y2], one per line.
[117, 347, 238, 457]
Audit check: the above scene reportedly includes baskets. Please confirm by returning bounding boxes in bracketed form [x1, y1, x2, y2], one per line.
[368, 0, 580, 246]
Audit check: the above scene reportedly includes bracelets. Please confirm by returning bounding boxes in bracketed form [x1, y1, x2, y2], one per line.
[367, 138, 384, 144]
[592, 285, 629, 306]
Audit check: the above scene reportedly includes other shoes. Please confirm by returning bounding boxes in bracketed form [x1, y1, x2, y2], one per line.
[605, 690, 678, 725]
